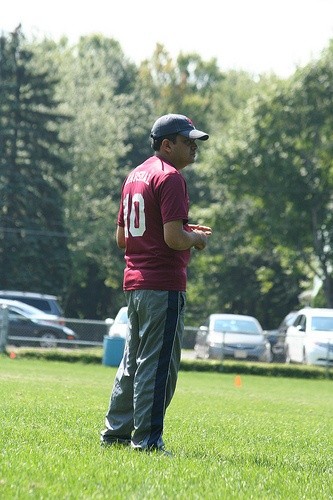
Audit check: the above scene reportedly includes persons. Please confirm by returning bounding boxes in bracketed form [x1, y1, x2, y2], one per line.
[99, 114, 212, 456]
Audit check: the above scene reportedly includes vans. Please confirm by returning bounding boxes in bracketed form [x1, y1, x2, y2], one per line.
[0, 290, 64, 317]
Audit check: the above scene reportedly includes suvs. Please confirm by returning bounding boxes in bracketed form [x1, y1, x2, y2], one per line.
[285, 307, 333, 367]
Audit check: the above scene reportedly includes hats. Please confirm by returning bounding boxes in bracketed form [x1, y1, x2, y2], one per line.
[150, 113, 210, 140]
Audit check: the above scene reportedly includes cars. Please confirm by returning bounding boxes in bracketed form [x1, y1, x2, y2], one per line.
[194, 313, 272, 362]
[105, 306, 131, 340]
[273, 311, 296, 361]
[0, 305, 79, 348]
[0, 299, 65, 326]
